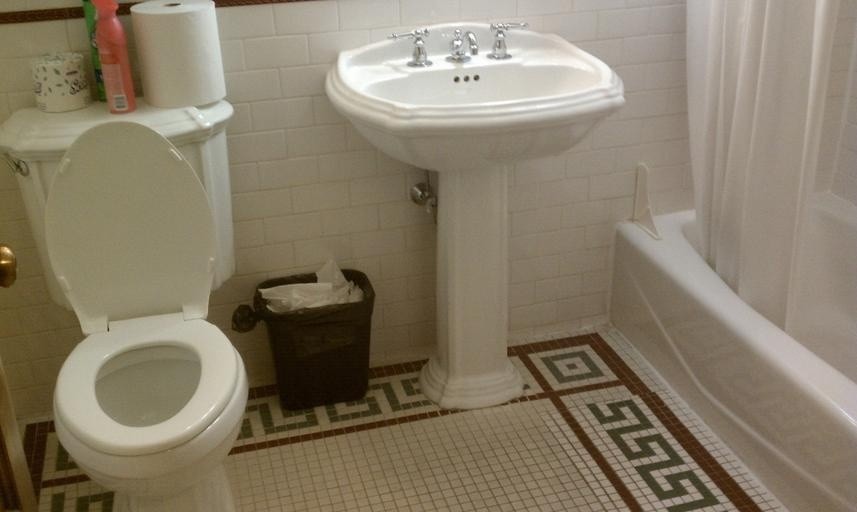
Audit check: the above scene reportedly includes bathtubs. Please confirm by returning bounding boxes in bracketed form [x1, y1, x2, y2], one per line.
[611, 192, 855, 512]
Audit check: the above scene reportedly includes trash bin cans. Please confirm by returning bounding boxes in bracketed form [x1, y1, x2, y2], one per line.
[254, 269, 378, 410]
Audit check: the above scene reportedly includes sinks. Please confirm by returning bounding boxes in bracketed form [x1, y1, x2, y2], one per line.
[324, 20, 625, 170]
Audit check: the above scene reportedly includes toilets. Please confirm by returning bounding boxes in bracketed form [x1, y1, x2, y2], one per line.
[1, 103, 249, 511]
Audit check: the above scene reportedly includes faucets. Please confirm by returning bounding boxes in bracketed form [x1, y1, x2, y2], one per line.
[449, 30, 478, 60]
